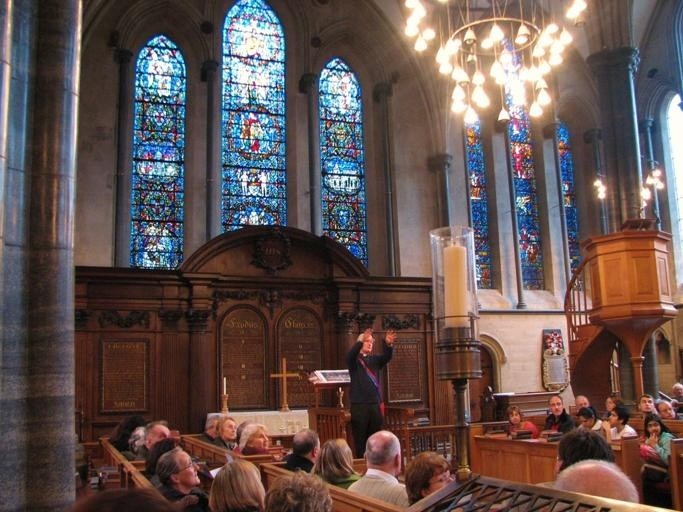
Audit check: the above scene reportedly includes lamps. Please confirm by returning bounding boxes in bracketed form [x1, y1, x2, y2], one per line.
[640, 161, 666, 207]
[405, 0, 587, 124]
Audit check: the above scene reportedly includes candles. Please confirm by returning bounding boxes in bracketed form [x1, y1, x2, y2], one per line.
[339, 386, 342, 393]
[442, 246, 470, 327]
[224, 377, 226, 395]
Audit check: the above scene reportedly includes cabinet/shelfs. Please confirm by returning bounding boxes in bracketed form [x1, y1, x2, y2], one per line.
[493, 390, 560, 421]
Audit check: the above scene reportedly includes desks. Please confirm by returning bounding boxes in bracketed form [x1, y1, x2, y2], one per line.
[473, 434, 644, 504]
[522, 416, 683, 438]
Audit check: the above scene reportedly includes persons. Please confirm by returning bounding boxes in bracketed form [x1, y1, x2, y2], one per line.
[234, 419, 252, 455]
[672, 382, 683, 413]
[543, 395, 575, 435]
[142, 438, 213, 486]
[263, 471, 334, 512]
[212, 416, 237, 451]
[206, 459, 266, 511]
[602, 394, 624, 421]
[157, 447, 208, 508]
[574, 394, 598, 419]
[200, 414, 224, 443]
[546, 458, 641, 512]
[638, 413, 677, 503]
[106, 415, 145, 449]
[312, 437, 363, 489]
[121, 427, 145, 459]
[238, 422, 279, 461]
[542, 331, 566, 353]
[404, 451, 473, 510]
[281, 427, 321, 474]
[346, 429, 412, 508]
[600, 405, 638, 451]
[504, 428, 617, 512]
[135, 420, 172, 459]
[639, 393, 658, 421]
[506, 406, 539, 439]
[654, 400, 682, 420]
[345, 327, 398, 459]
[575, 406, 603, 434]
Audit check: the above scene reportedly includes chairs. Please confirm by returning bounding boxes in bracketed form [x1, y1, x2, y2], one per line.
[308, 382, 415, 475]
[99, 437, 146, 472]
[259, 457, 405, 512]
[120, 462, 169, 505]
[182, 433, 273, 470]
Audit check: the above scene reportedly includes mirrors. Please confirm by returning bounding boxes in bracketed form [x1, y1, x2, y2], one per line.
[543, 356, 569, 392]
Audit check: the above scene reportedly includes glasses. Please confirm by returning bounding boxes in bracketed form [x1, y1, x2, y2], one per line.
[429, 474, 451, 485]
[177, 462, 193, 474]
